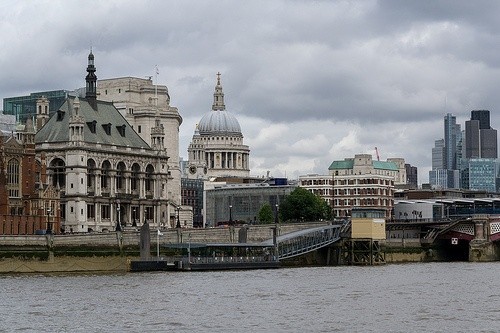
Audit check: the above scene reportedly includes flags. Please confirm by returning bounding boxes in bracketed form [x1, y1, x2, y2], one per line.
[156, 66, 159, 74]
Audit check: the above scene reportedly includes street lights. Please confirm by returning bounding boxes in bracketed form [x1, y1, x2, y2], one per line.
[228, 205, 233, 226]
[132, 209, 137, 226]
[144, 209, 147, 223]
[115, 207, 121, 231]
[175, 206, 181, 228]
[46, 208, 51, 234]
[275, 204, 279, 223]
